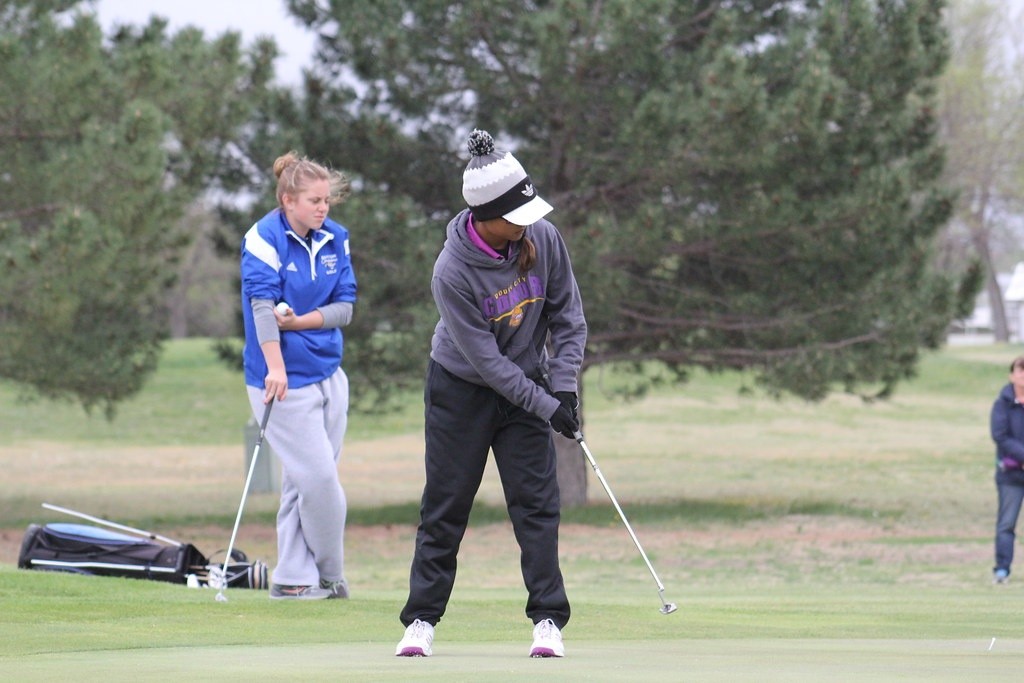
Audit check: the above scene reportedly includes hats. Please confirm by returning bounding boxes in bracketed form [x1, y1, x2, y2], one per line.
[462, 129, 554, 226]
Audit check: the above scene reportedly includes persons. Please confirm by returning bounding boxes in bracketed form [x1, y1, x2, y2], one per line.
[990, 356, 1024, 584]
[243, 153, 357, 599]
[395, 128, 588, 656]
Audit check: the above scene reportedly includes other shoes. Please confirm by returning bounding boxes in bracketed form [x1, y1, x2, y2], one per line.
[996, 569, 1008, 583]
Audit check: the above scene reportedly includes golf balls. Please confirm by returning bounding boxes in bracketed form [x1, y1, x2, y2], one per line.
[276, 302, 290, 316]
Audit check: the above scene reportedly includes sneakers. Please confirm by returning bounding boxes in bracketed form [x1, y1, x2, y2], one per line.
[319, 579, 348, 599]
[269, 583, 332, 599]
[396, 619, 435, 657]
[529, 618, 564, 658]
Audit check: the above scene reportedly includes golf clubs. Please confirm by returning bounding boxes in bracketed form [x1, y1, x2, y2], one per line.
[535, 363, 678, 615]
[207, 394, 275, 589]
[183, 564, 236, 581]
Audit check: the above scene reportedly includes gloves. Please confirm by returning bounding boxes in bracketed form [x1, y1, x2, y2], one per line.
[555, 392, 579, 420]
[548, 406, 579, 439]
